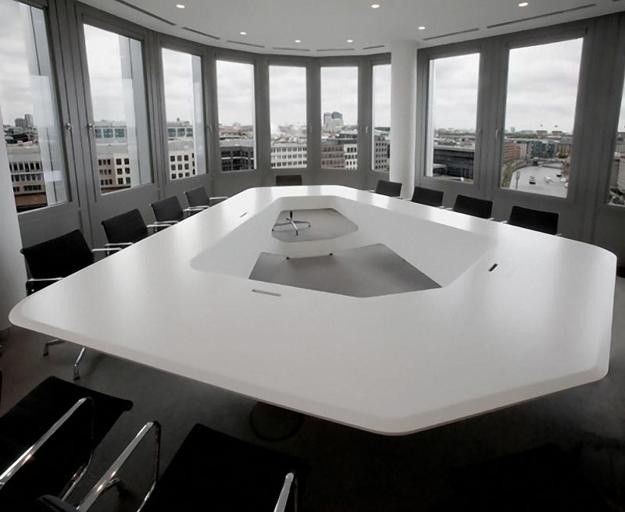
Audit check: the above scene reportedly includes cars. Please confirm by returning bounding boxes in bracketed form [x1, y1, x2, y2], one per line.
[556, 174, 568, 188]
[529, 176, 536, 184]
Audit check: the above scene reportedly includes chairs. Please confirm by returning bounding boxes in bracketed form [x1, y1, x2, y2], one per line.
[37, 422, 304, 511]
[2, 375, 137, 510]
[369, 179, 559, 235]
[18, 201, 124, 378]
[149, 195, 203, 233]
[185, 186, 229, 217]
[99, 208, 173, 257]
[272, 173, 311, 236]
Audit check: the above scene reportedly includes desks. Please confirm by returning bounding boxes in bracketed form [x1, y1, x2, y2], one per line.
[9, 184, 619, 442]
